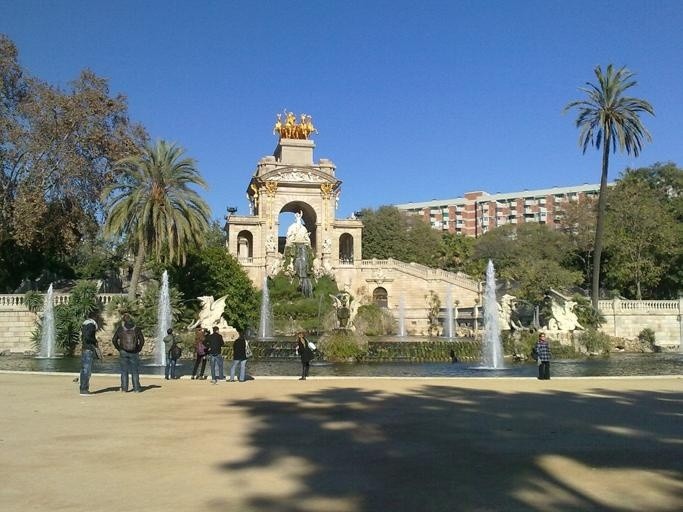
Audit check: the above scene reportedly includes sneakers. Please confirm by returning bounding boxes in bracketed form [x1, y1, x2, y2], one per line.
[165, 377, 244, 384]
[134, 387, 143, 392]
[80, 391, 96, 397]
[299, 378, 306, 381]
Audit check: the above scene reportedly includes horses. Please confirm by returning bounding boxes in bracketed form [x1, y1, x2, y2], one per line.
[273, 113, 319, 141]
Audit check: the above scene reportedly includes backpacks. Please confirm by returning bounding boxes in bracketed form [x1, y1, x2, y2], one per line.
[196, 342, 208, 356]
[122, 325, 137, 352]
[245, 340, 253, 358]
[168, 336, 181, 360]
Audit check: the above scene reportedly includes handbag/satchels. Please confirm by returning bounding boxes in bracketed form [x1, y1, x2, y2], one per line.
[93, 348, 103, 360]
[308, 342, 316, 351]
[531, 347, 538, 361]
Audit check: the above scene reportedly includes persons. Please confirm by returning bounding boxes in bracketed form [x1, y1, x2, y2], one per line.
[202, 326, 226, 383]
[78, 311, 98, 396]
[192, 328, 210, 379]
[112, 314, 145, 393]
[296, 332, 314, 381]
[295, 209, 304, 230]
[227, 330, 249, 382]
[162, 328, 182, 380]
[534, 332, 551, 381]
